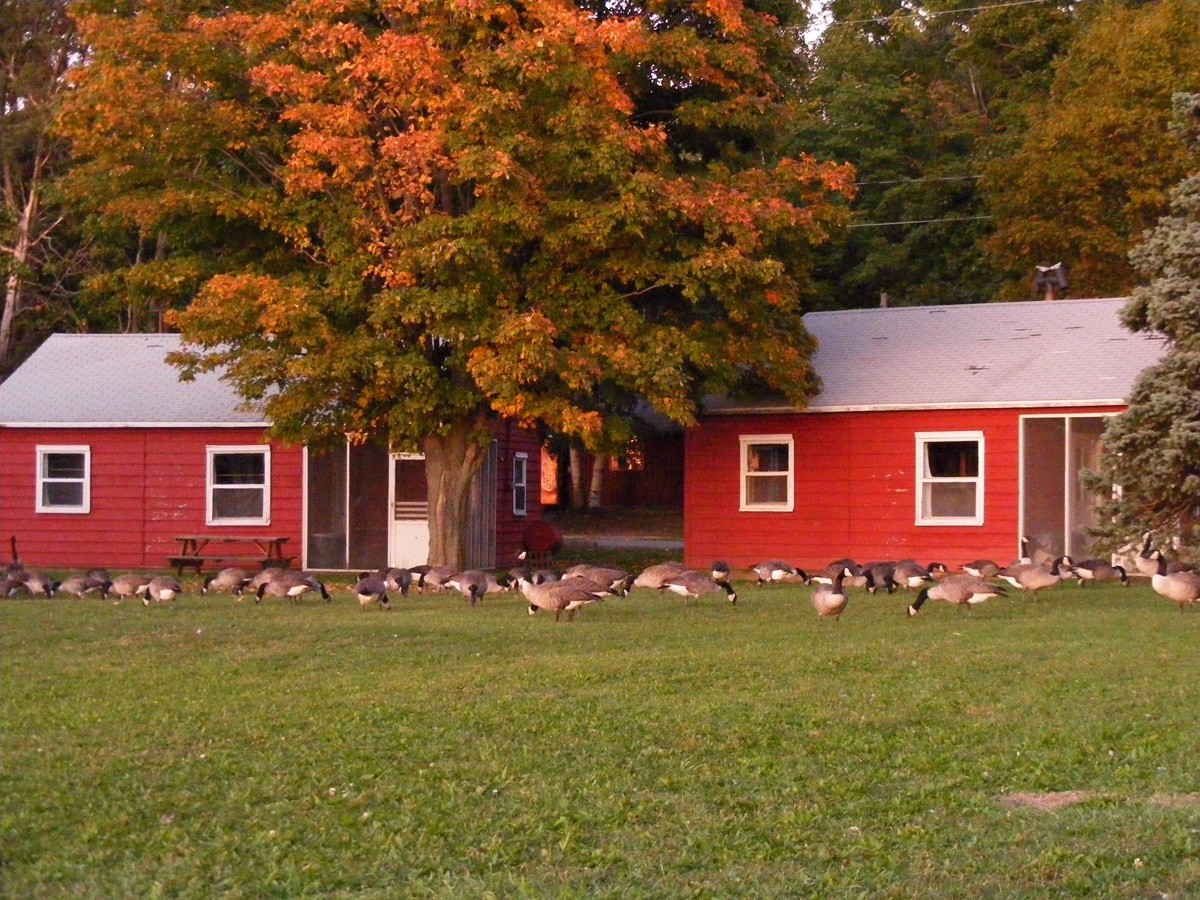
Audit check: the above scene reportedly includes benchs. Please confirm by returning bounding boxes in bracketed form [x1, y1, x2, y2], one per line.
[166, 555, 297, 562]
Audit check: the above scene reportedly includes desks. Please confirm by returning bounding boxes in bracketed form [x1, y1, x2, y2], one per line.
[175, 535, 289, 575]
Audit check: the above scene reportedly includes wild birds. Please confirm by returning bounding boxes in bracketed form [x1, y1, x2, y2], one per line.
[0, 537, 1200, 627]
